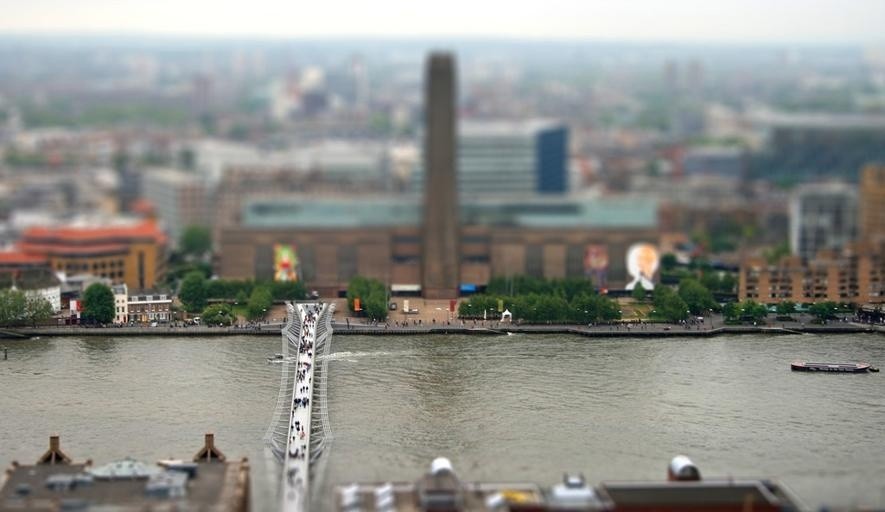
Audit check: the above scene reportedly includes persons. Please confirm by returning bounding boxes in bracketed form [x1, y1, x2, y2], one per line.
[504, 303, 885, 331]
[85, 314, 289, 330]
[300, 315, 502, 329]
[284, 299, 323, 511]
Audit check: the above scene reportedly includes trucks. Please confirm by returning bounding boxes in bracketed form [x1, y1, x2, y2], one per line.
[184, 316, 201, 325]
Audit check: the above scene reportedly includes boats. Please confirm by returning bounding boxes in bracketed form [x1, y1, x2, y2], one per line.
[268, 352, 283, 361]
[791, 361, 879, 373]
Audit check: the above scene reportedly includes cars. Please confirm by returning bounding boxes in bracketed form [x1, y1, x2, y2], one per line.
[694, 317, 704, 325]
[409, 308, 418, 314]
